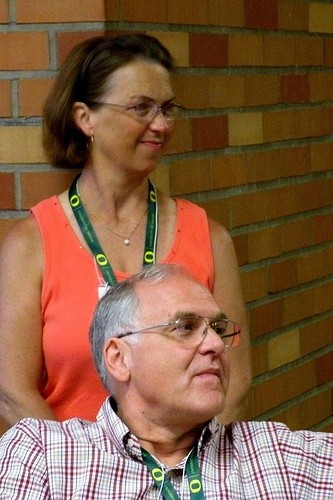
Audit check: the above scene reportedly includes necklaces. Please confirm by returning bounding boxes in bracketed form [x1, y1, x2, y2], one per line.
[73, 171, 156, 245]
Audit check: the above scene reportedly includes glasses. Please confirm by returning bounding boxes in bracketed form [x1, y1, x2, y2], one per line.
[88, 99, 187, 121]
[118, 315, 240, 348]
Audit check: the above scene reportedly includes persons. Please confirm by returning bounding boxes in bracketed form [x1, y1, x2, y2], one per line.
[0, 27, 256, 426]
[1, 264, 333, 500]
